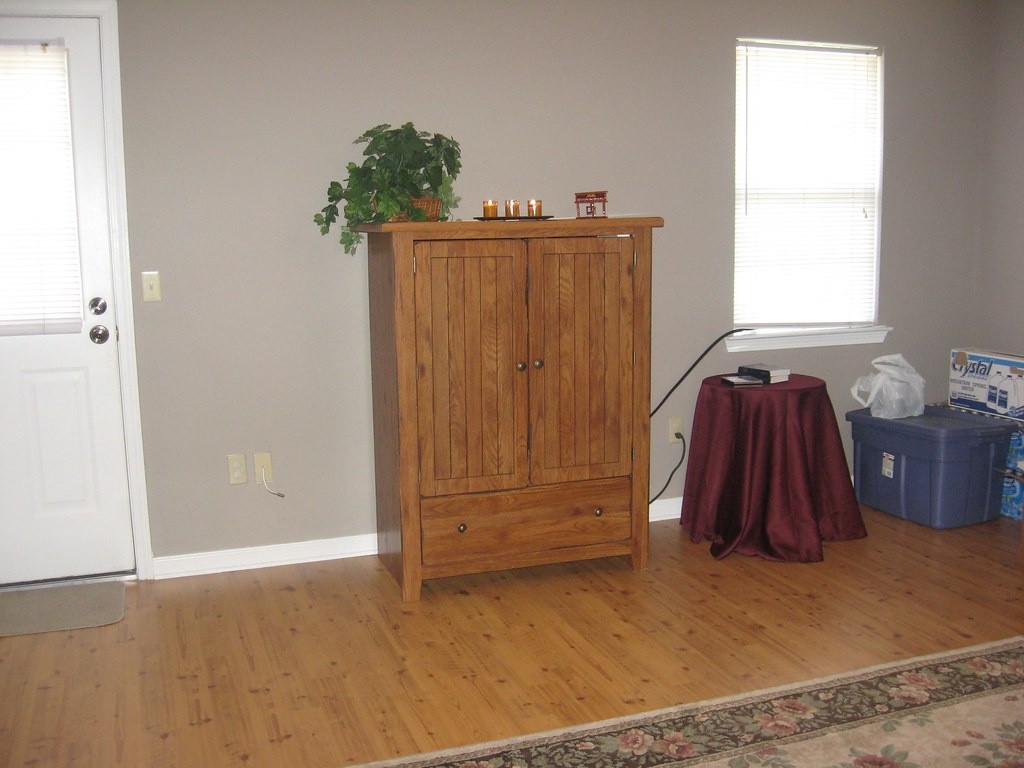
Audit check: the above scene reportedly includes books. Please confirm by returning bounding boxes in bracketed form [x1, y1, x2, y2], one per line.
[721, 375, 763, 389]
[738, 363, 791, 384]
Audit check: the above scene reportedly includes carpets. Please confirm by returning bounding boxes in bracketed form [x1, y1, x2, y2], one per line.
[0, 581, 128, 638]
[352, 633, 1024, 768]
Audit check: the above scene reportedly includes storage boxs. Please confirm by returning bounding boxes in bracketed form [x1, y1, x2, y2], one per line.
[948, 346, 1024, 421]
[845, 404, 1019, 529]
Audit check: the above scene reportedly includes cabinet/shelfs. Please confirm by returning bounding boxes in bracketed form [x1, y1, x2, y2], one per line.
[347, 218, 664, 602]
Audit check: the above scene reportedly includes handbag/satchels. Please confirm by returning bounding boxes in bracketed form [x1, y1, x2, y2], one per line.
[850, 354, 926, 419]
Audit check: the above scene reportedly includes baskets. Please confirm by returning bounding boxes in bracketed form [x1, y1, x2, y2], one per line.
[372, 195, 442, 223]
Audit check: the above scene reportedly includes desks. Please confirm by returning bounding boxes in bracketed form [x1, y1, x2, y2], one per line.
[679, 373, 869, 564]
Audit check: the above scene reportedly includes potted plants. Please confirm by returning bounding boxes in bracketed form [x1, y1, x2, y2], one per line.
[312, 121, 462, 255]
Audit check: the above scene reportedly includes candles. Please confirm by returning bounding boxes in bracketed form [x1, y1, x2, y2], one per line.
[505, 199, 519, 217]
[528, 199, 542, 217]
[483, 200, 498, 217]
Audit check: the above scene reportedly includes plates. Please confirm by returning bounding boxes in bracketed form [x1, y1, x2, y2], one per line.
[473, 216, 554, 220]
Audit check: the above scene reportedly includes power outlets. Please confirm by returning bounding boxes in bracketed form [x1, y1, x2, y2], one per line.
[669, 417, 684, 442]
[227, 455, 247, 484]
